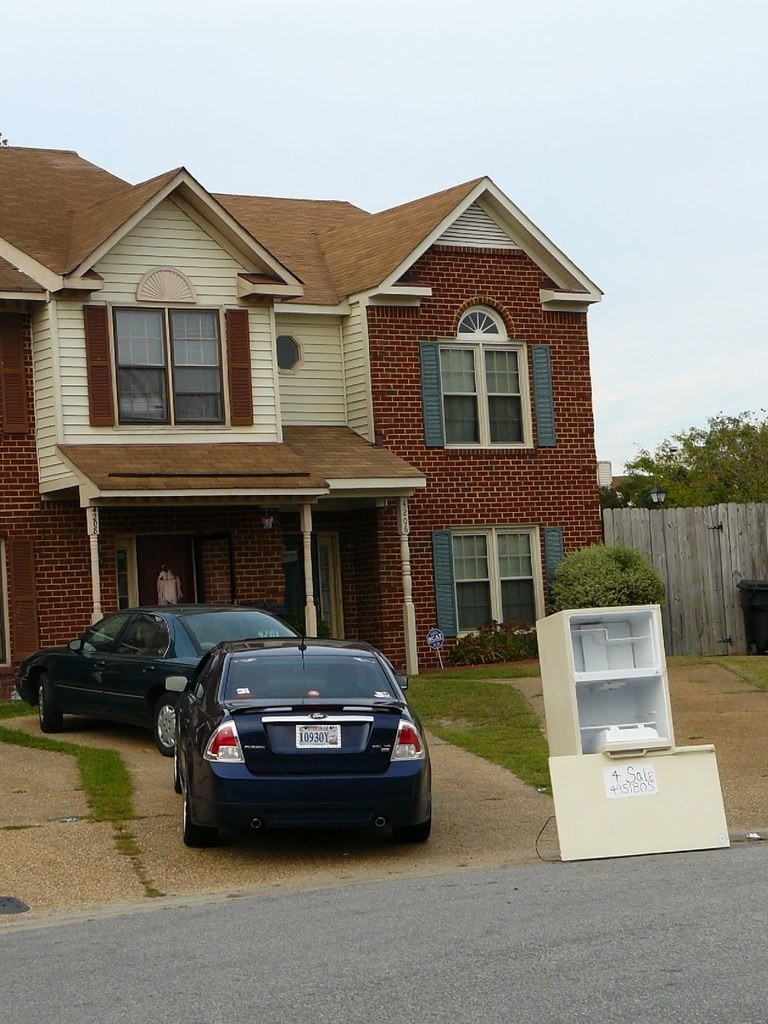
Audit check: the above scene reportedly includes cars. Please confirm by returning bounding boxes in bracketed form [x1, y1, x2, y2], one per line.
[14, 602, 305, 758]
[170, 634, 435, 851]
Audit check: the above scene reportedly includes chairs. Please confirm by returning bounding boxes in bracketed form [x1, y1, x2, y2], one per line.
[326, 663, 361, 689]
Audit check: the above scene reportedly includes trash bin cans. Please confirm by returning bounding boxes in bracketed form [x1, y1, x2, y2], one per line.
[735, 579, 768, 655]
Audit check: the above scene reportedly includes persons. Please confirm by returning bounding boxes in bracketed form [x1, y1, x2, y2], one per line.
[157, 563, 184, 606]
